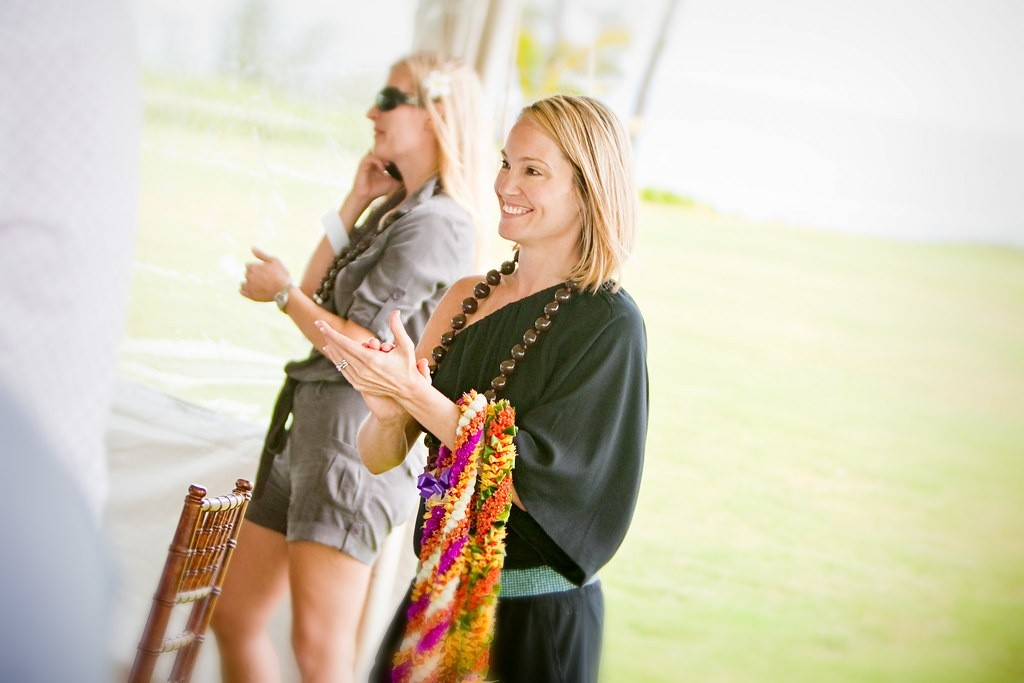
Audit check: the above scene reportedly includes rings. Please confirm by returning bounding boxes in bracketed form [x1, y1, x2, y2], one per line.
[337, 360, 349, 371]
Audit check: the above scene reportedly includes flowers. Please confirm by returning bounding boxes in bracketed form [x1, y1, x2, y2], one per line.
[387, 388, 518, 683]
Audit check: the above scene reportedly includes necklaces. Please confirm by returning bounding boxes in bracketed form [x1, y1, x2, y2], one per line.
[424, 251, 579, 448]
[313, 201, 405, 304]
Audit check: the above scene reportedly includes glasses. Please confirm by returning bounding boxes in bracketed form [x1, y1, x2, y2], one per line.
[376, 86, 439, 112]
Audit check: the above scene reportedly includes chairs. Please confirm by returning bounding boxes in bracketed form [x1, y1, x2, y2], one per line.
[129, 478, 254, 683]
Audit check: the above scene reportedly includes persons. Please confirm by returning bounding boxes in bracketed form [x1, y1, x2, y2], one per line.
[314, 95, 649, 683]
[209, 52, 484, 683]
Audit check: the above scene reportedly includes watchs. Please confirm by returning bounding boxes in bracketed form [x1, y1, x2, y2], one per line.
[274, 284, 295, 312]
